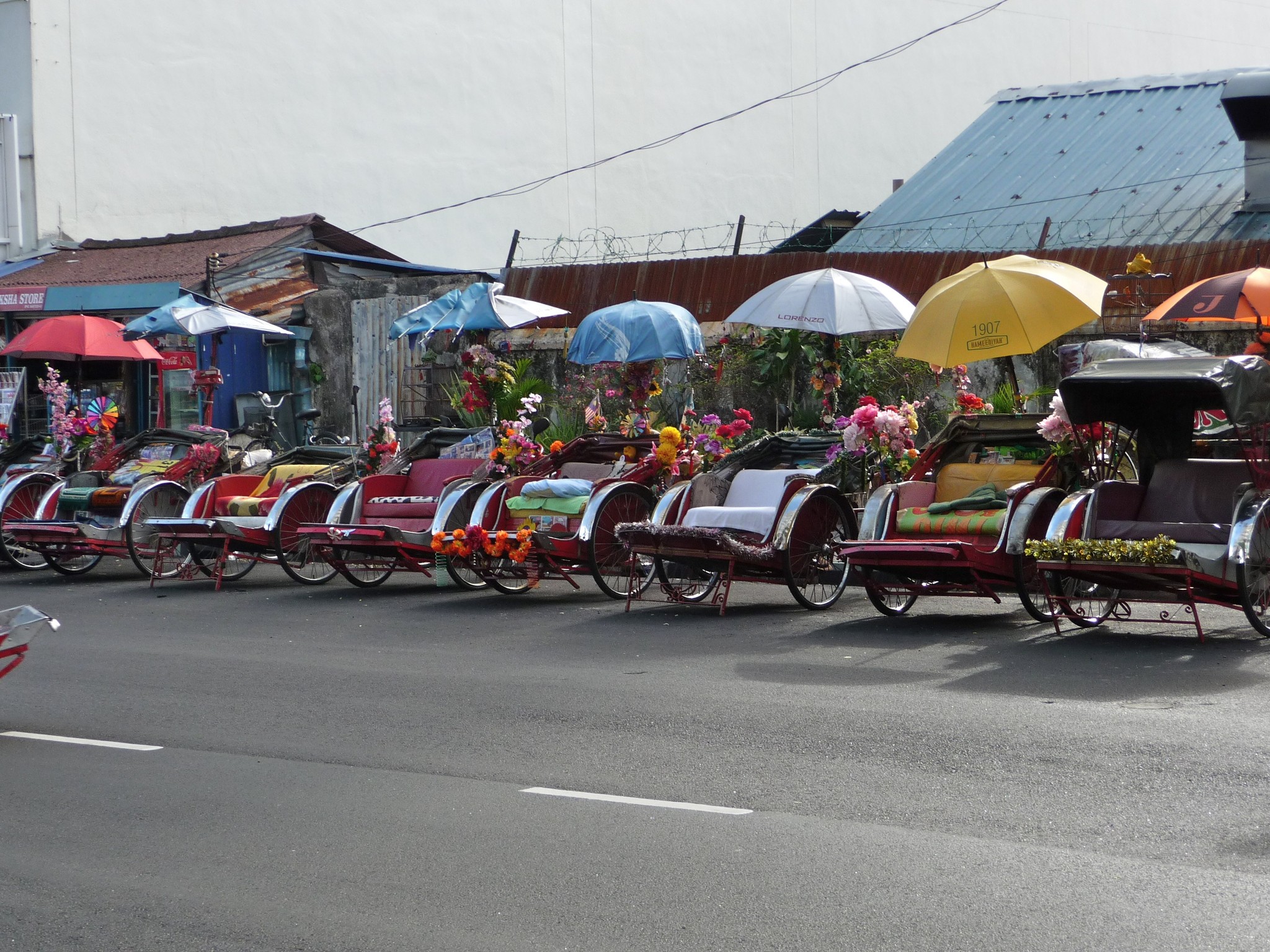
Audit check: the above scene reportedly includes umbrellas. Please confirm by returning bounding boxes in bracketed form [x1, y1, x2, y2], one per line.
[720, 256, 917, 339]
[386, 281, 572, 346]
[893, 250, 1112, 414]
[565, 289, 707, 365]
[114, 288, 296, 426]
[0, 306, 163, 421]
[1137, 248, 1270, 326]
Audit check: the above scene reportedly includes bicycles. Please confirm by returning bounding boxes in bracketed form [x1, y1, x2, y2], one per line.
[245, 389, 347, 458]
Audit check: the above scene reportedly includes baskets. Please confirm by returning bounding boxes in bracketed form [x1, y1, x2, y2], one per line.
[244, 406, 280, 439]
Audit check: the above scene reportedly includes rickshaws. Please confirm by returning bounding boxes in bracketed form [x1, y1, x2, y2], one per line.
[0, 354, 1269, 646]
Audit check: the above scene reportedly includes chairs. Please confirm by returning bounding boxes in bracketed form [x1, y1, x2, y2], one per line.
[55, 438, 200, 525]
[681, 465, 818, 539]
[895, 460, 1049, 535]
[358, 455, 488, 531]
[504, 462, 619, 543]
[1103, 455, 1255, 543]
[211, 464, 337, 529]
